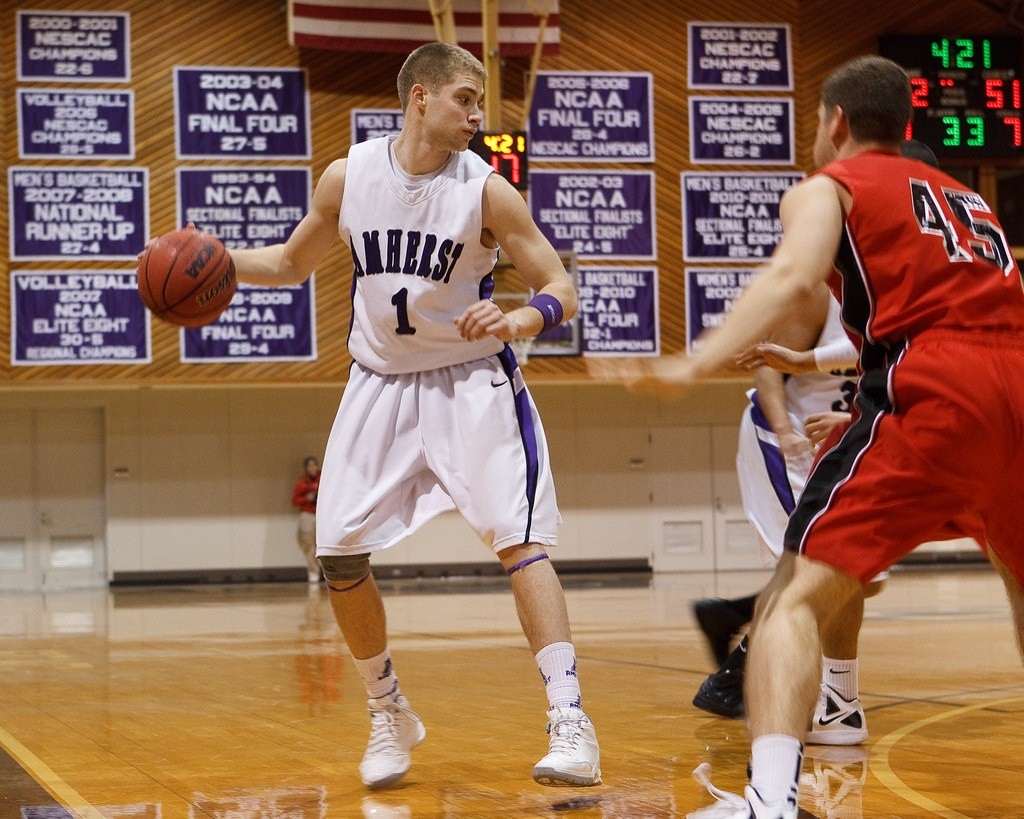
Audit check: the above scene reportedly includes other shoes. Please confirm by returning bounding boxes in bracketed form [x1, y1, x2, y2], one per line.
[307, 569, 320, 583]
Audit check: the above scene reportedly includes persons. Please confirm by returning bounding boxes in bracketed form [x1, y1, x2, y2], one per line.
[292, 457, 321, 582]
[648, 58, 1024, 819]
[136, 43, 602, 787]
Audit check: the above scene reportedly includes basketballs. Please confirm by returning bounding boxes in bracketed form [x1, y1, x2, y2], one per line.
[136, 229, 238, 329]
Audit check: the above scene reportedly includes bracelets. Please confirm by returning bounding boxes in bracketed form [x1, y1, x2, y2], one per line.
[527, 293, 563, 336]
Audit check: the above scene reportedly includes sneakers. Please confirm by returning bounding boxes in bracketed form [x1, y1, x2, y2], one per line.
[358, 693, 427, 789]
[686, 762, 799, 819]
[805, 685, 868, 745]
[693, 674, 745, 718]
[531, 707, 603, 788]
[693, 597, 750, 668]
